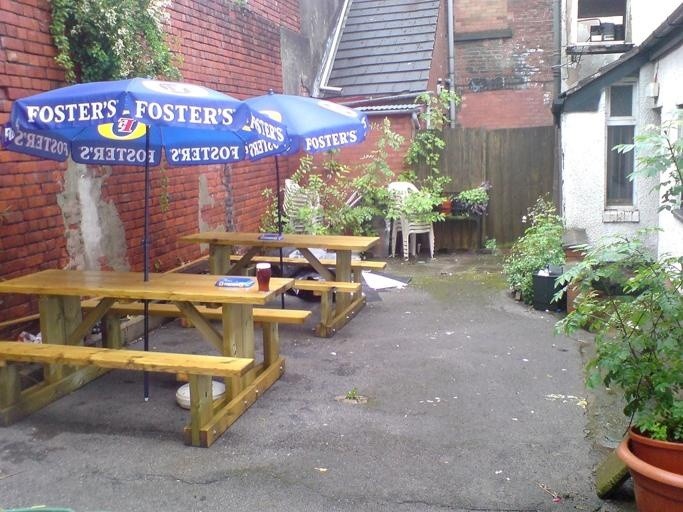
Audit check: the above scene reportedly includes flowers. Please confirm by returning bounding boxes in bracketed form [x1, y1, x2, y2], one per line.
[456, 178, 496, 217]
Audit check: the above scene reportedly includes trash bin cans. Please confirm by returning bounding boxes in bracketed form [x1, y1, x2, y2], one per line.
[532, 271, 564, 311]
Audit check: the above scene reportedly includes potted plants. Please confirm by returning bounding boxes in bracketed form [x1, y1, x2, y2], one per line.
[553, 225, 683, 512]
[438, 195, 453, 215]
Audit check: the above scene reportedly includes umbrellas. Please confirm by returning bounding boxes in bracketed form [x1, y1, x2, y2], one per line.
[0, 75, 292, 278]
[240, 86, 371, 235]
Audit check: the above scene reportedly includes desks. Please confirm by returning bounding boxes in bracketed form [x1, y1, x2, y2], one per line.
[179, 230, 383, 315]
[0, 269, 295, 393]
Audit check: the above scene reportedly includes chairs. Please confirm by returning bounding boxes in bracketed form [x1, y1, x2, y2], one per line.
[384, 179, 435, 262]
[282, 177, 326, 234]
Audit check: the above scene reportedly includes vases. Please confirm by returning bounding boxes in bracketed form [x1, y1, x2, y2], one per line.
[532, 263, 604, 325]
[452, 202, 467, 216]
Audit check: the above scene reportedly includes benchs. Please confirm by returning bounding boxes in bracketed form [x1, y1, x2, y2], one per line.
[1, 340, 256, 446]
[230, 255, 387, 293]
[294, 279, 362, 337]
[77, 299, 311, 368]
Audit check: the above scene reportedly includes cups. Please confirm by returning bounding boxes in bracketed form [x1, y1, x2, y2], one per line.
[256, 263, 272, 292]
